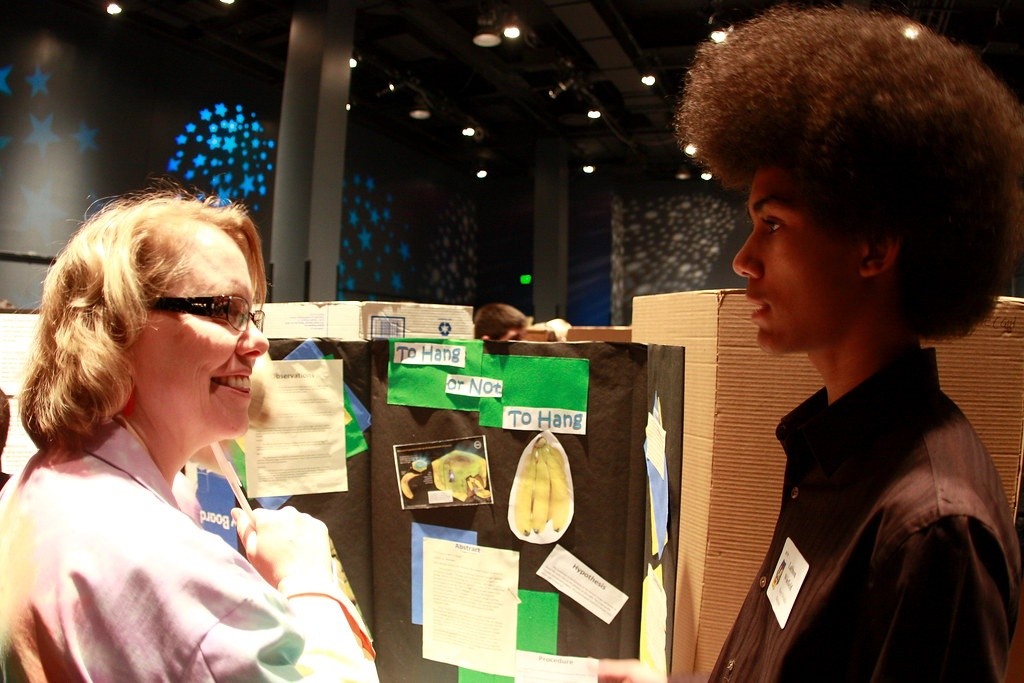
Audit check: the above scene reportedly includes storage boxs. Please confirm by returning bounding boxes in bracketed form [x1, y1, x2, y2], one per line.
[633, 291, 1024, 683]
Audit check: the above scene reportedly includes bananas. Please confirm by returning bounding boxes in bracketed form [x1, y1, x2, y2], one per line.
[515, 436, 568, 537]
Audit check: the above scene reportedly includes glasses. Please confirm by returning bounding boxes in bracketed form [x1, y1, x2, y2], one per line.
[144, 295, 265, 333]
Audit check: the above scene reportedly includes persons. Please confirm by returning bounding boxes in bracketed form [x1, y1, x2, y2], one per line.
[473, 303, 534, 341]
[0, 192, 380, 683]
[588, 5, 1024, 683]
